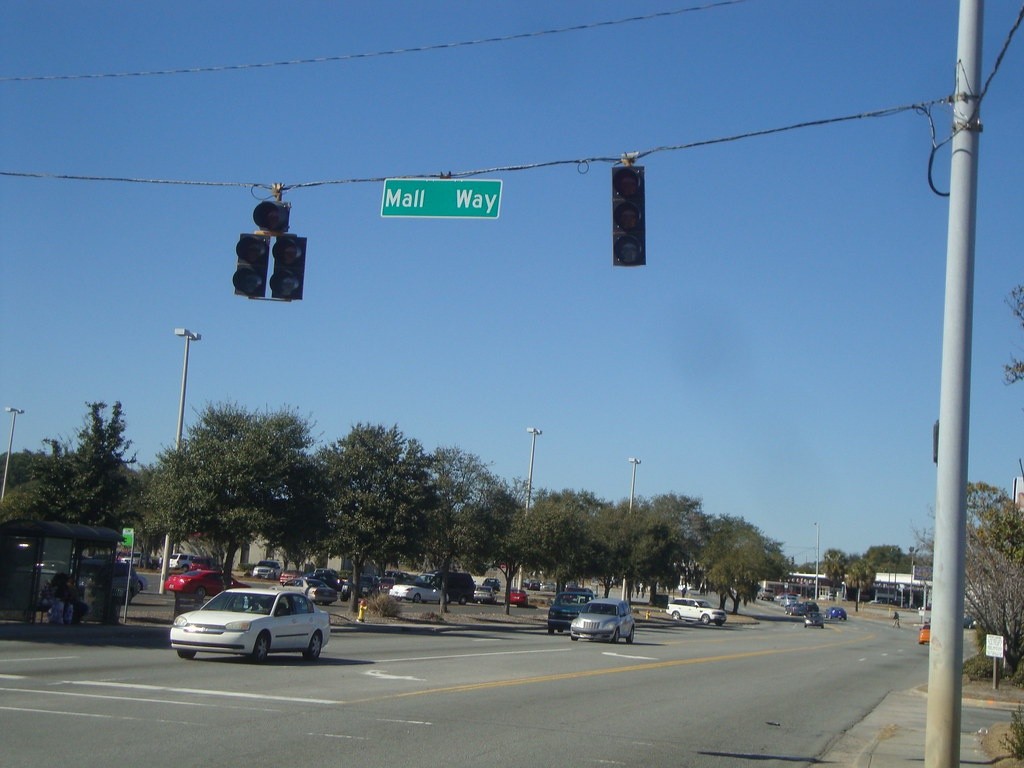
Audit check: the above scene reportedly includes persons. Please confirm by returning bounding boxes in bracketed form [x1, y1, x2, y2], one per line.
[892, 611, 900, 629]
[69, 580, 88, 625]
[259, 598, 280, 614]
[117, 552, 121, 561]
[44, 570, 73, 625]
[682, 588, 686, 598]
[158, 554, 163, 569]
[268, 569, 276, 580]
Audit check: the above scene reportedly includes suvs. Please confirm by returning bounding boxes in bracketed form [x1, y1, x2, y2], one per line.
[665, 598, 727, 627]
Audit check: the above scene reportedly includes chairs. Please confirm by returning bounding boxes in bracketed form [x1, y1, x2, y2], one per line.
[606, 605, 619, 614]
[589, 604, 602, 613]
[232, 597, 250, 610]
[562, 598, 570, 603]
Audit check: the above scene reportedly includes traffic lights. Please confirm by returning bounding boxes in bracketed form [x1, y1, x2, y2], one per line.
[251, 200, 293, 232]
[268, 235, 307, 300]
[231, 233, 270, 298]
[612, 163, 647, 267]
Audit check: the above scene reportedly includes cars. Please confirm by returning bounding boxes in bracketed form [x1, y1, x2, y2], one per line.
[569, 598, 636, 644]
[279, 570, 303, 584]
[252, 559, 283, 578]
[81, 550, 218, 604]
[473, 585, 498, 604]
[282, 567, 477, 604]
[163, 568, 252, 598]
[505, 589, 530, 608]
[802, 612, 825, 629]
[481, 578, 501, 592]
[918, 625, 930, 645]
[523, 579, 599, 634]
[963, 615, 981, 629]
[756, 587, 820, 616]
[825, 606, 847, 621]
[169, 586, 332, 666]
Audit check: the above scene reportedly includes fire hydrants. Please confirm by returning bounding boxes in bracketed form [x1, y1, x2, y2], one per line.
[645, 610, 653, 622]
[356, 597, 369, 622]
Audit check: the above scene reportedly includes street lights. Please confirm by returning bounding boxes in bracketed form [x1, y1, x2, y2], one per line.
[908, 545, 921, 612]
[621, 458, 641, 599]
[154, 329, 200, 593]
[517, 428, 543, 592]
[1, 406, 25, 503]
[812, 521, 820, 600]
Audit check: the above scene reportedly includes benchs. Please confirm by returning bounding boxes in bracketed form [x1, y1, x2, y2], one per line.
[27, 602, 50, 624]
